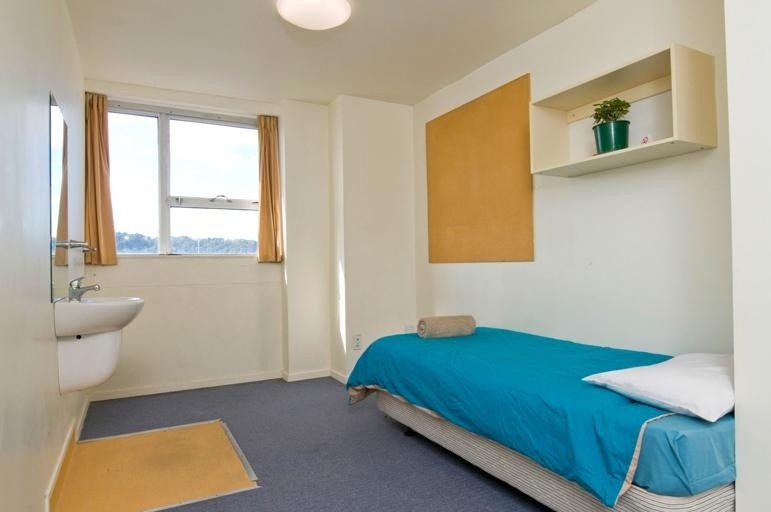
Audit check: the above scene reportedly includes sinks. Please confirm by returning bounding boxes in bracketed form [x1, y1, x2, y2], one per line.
[54, 296, 144, 338]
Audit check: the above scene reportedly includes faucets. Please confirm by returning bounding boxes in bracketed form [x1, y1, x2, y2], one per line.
[66, 273, 100, 302]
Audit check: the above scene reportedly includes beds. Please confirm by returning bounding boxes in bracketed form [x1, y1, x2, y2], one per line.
[345, 326, 736, 511]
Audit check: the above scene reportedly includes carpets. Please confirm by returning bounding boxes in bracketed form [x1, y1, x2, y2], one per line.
[76, 377, 453, 512]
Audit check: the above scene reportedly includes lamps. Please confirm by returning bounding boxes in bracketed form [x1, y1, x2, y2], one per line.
[277, 0, 352, 30]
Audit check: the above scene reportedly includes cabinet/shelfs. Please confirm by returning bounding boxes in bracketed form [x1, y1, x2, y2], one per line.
[529, 43, 718, 175]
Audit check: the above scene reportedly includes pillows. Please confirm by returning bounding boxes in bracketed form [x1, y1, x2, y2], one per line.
[582, 352, 735, 422]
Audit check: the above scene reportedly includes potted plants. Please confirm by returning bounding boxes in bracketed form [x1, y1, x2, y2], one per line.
[592, 97, 631, 154]
